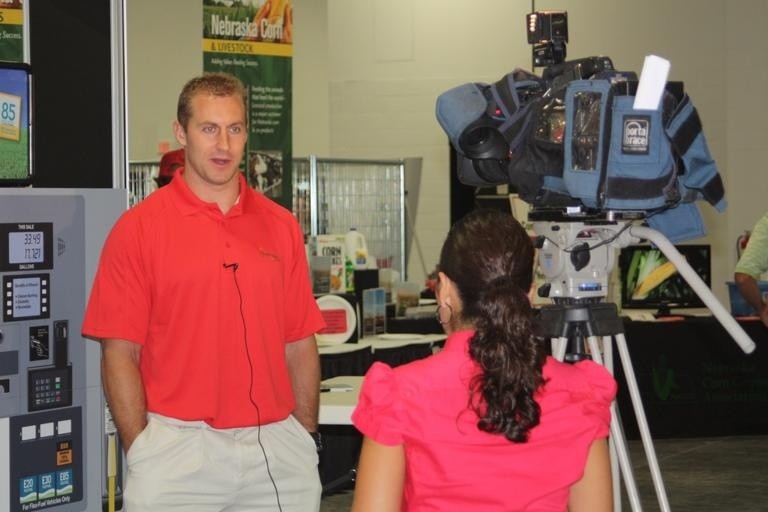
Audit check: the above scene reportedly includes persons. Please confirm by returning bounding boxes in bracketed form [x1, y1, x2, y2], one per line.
[81, 72, 325, 511]
[351, 208, 618, 512]
[735, 213, 768, 329]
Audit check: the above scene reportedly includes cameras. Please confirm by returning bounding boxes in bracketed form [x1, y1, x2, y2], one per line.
[435, 12, 728, 240]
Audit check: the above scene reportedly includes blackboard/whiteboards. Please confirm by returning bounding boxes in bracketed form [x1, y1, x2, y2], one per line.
[0, 1, 113, 189]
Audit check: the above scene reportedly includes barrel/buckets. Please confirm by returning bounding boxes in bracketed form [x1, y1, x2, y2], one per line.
[344, 227, 369, 270]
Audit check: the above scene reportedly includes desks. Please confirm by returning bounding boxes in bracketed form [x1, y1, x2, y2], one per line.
[317, 375, 367, 425]
[615, 314, 766, 441]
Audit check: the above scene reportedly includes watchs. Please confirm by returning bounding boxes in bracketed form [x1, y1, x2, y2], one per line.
[310, 433, 325, 451]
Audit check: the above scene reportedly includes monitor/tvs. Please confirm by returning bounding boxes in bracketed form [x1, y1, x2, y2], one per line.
[619, 245, 712, 318]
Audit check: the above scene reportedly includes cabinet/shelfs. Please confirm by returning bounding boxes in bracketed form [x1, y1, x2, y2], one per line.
[317, 344, 372, 496]
[362, 337, 447, 370]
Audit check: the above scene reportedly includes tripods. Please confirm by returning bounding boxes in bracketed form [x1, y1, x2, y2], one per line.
[528, 209, 755, 512]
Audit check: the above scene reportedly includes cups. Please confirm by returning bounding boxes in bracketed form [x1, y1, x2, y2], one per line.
[395, 280, 419, 317]
